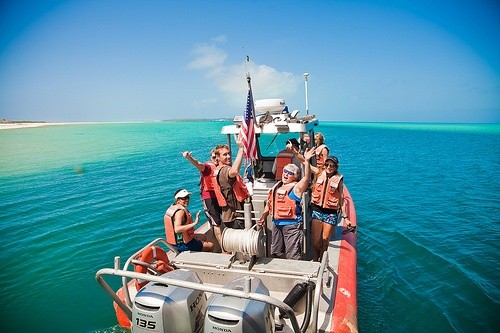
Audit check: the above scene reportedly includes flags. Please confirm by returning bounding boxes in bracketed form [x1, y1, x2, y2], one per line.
[239, 89, 258, 166]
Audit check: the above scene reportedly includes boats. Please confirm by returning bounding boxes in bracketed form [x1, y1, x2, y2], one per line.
[94, 71, 359, 333]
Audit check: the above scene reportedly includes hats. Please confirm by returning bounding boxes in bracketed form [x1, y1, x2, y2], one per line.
[286, 138, 300, 146]
[174, 188, 192, 200]
[325, 155, 340, 167]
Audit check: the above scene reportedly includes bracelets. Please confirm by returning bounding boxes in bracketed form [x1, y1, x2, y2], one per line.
[194, 221, 197, 225]
[343, 216, 348, 218]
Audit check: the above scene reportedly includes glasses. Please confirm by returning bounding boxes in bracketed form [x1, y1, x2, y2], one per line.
[179, 195, 189, 200]
[283, 168, 294, 175]
[211, 155, 217, 159]
[324, 163, 336, 168]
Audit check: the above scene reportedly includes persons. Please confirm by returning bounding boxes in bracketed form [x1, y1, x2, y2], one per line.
[211, 134, 250, 229]
[298, 131, 311, 146]
[180, 151, 231, 254]
[301, 120, 308, 124]
[164, 189, 214, 252]
[271, 139, 300, 182]
[256, 147, 316, 260]
[286, 140, 351, 267]
[314, 132, 329, 167]
[244, 161, 254, 184]
